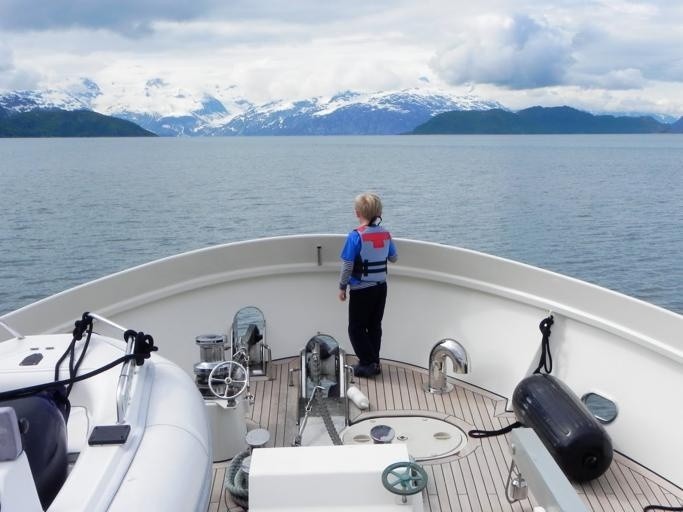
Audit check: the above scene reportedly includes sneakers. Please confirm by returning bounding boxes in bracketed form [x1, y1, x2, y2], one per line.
[351, 363, 380, 378]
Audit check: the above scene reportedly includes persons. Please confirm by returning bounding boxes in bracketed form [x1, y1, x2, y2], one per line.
[337, 192, 398, 379]
